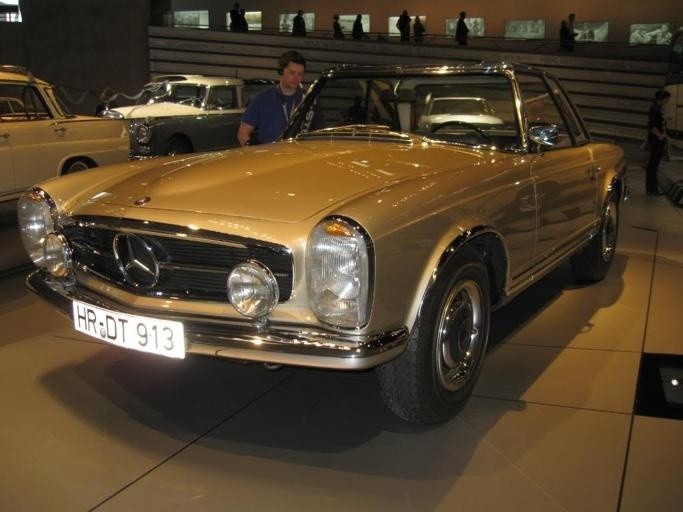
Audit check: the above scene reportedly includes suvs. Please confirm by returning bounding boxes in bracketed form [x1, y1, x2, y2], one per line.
[0, 64, 134, 204]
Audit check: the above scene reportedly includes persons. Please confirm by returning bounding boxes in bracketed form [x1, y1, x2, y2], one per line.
[331, 12, 345, 38]
[575, 21, 595, 40]
[347, 94, 367, 123]
[644, 23, 671, 45]
[291, 9, 307, 37]
[238, 8, 248, 32]
[567, 11, 578, 52]
[350, 12, 366, 41]
[229, 2, 242, 33]
[629, 24, 652, 43]
[453, 10, 470, 47]
[411, 14, 426, 44]
[643, 87, 672, 196]
[93, 86, 119, 117]
[394, 9, 410, 43]
[556, 20, 573, 51]
[234, 48, 323, 148]
